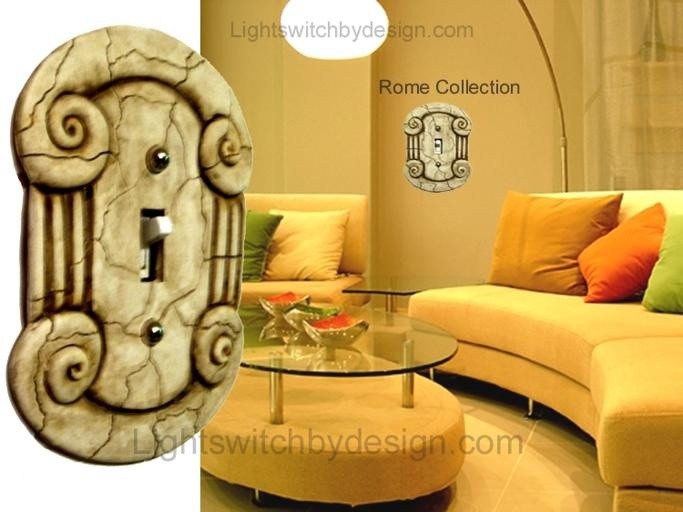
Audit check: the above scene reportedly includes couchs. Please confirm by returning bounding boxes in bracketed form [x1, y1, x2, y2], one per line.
[230, 190, 369, 298]
[400, 186, 683, 512]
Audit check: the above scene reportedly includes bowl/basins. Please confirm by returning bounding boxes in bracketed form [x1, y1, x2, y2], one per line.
[282, 304, 340, 332]
[284, 332, 321, 360]
[259, 292, 311, 315]
[308, 346, 373, 373]
[257, 317, 299, 343]
[302, 313, 369, 346]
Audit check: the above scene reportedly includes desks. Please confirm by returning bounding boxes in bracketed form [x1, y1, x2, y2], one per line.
[337, 271, 424, 309]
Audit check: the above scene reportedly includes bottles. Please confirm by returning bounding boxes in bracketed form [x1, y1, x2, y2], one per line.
[640, 1, 667, 63]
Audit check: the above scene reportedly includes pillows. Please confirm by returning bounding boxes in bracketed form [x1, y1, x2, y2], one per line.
[639, 207, 683, 314]
[263, 205, 350, 281]
[488, 184, 625, 302]
[572, 200, 667, 305]
[238, 207, 284, 282]
